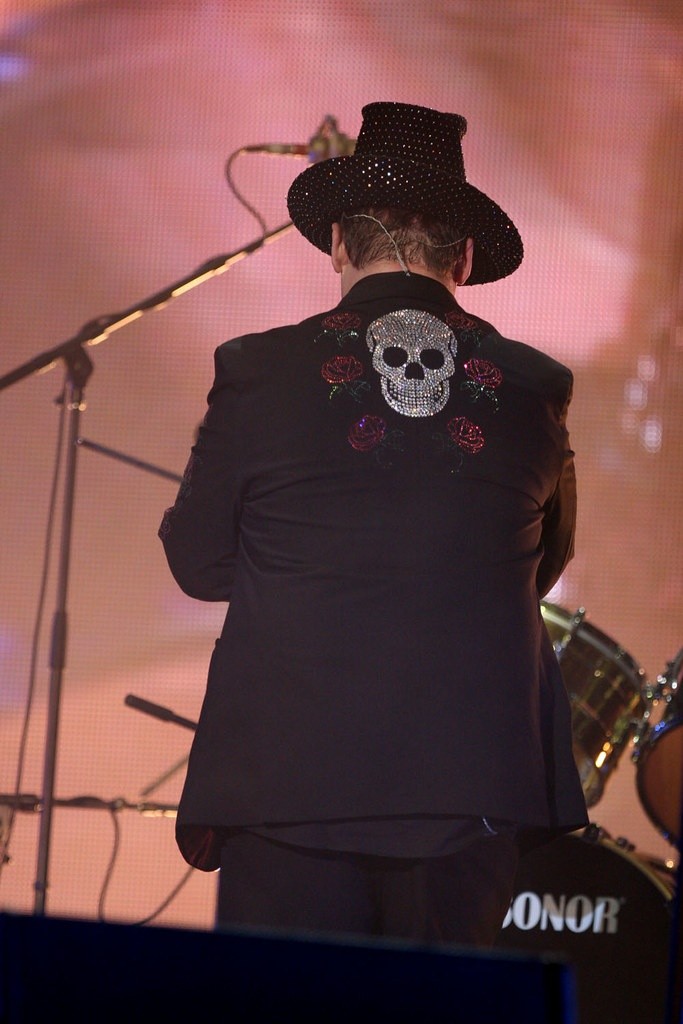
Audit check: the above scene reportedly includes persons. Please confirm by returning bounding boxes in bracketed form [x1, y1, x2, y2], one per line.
[159, 101, 586, 951]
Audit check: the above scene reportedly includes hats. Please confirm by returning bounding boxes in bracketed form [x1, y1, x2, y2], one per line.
[285, 101, 524, 287]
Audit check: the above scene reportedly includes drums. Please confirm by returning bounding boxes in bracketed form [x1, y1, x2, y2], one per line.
[484, 603, 683, 1024]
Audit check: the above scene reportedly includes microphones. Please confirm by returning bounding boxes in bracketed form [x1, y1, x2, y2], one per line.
[123, 694, 197, 731]
[242, 133, 358, 162]
